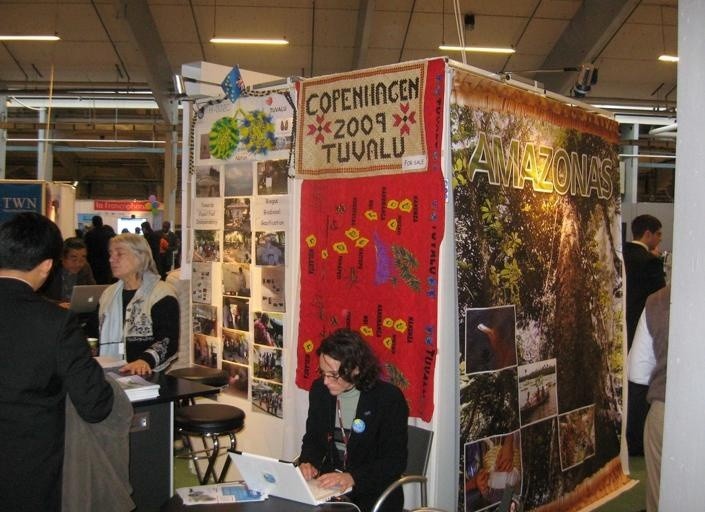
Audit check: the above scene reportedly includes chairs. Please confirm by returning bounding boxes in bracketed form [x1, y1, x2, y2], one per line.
[374, 424, 434, 511]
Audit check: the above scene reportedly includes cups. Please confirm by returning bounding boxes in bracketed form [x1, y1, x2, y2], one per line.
[87, 338, 98, 356]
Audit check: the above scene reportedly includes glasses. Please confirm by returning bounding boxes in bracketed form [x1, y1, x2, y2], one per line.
[316, 365, 353, 385]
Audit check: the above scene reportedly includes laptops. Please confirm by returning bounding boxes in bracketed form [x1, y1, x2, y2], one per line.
[68, 284, 113, 313]
[227, 449, 353, 506]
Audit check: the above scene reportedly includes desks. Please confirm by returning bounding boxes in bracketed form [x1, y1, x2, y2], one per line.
[128, 372, 220, 511]
[157, 480, 356, 512]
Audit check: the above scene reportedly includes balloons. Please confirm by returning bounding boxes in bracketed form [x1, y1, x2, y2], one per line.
[145, 195, 160, 216]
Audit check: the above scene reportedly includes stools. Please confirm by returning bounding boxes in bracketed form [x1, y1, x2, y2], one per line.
[167, 368, 229, 483]
[174, 404, 246, 485]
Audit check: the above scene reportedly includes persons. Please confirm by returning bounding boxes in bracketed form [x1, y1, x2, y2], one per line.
[466, 435, 513, 511]
[254, 313, 277, 347]
[300, 329, 408, 512]
[0, 215, 178, 512]
[254, 352, 283, 416]
[627, 283, 671, 512]
[622, 214, 667, 456]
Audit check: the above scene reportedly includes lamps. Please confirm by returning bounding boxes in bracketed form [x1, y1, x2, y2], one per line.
[0, 0, 64, 41]
[573, 61, 599, 99]
[656, 0, 679, 62]
[209, 0, 289, 46]
[439, 0, 517, 54]
